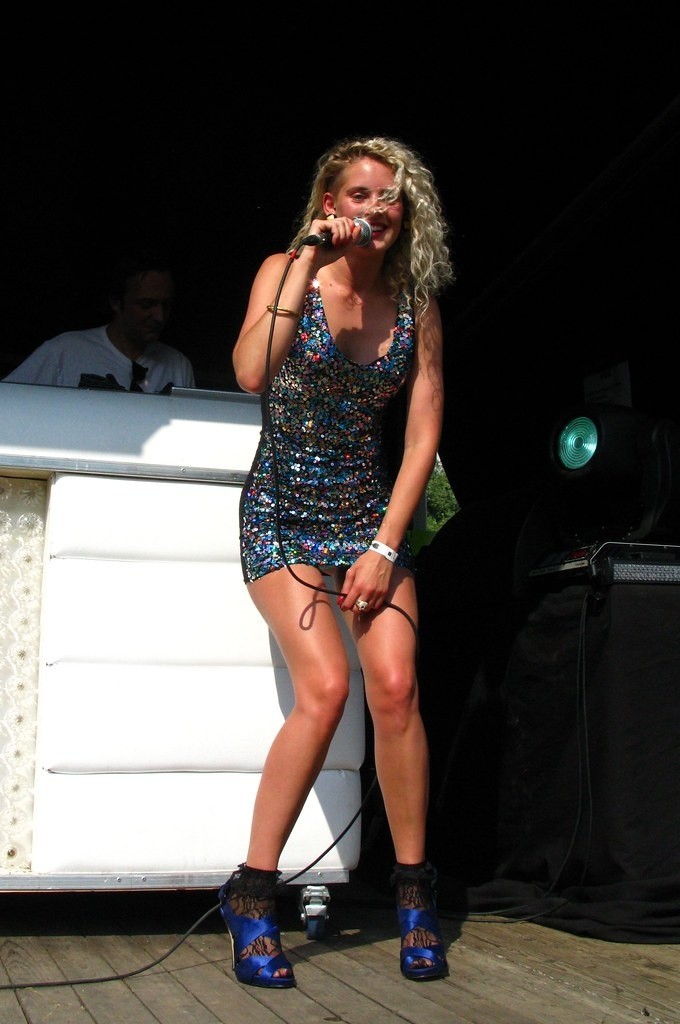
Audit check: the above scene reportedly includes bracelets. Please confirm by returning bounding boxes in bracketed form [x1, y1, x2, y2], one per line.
[266, 304, 301, 318]
[368, 539, 398, 562]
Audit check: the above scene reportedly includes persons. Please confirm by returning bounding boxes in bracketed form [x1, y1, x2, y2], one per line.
[219, 133, 452, 987]
[0, 255, 196, 395]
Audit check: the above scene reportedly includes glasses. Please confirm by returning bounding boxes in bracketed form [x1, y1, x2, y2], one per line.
[129, 360, 149, 392]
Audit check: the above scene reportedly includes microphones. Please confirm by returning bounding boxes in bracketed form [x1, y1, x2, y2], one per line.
[302, 219, 373, 247]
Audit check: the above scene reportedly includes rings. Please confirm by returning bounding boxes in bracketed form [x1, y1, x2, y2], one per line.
[355, 599, 368, 611]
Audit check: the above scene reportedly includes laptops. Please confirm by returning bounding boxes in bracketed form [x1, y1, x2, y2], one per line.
[170, 386, 261, 404]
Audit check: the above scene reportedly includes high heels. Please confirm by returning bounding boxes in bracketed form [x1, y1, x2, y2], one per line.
[398, 905, 449, 980]
[217, 884, 297, 990]
